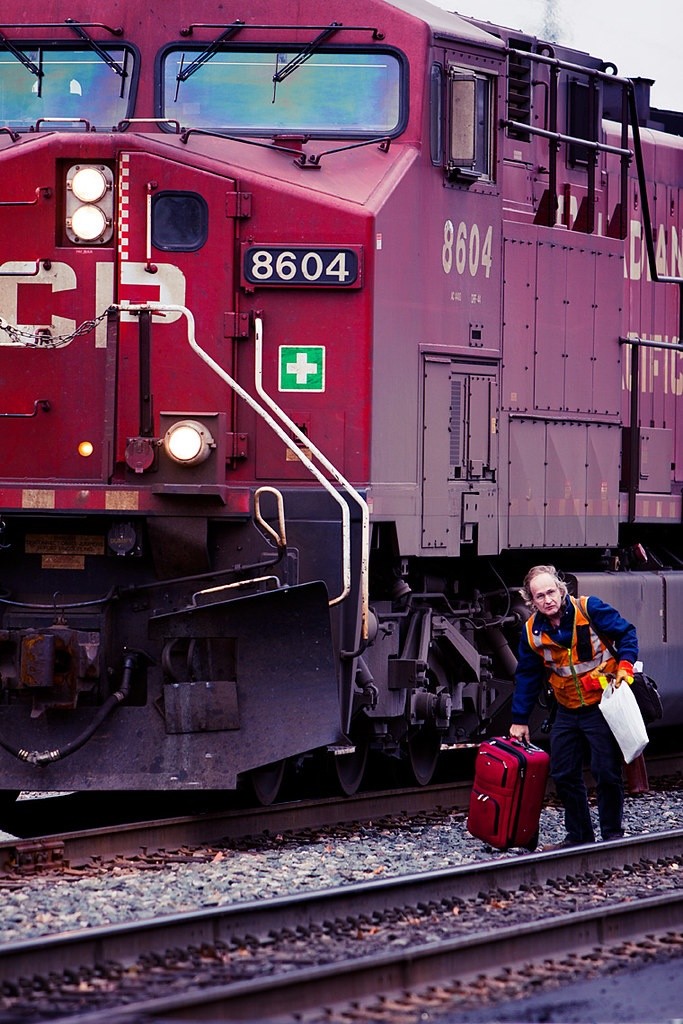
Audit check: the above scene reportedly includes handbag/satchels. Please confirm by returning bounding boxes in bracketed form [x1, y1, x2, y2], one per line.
[598, 678, 650, 765]
[631, 671, 663, 727]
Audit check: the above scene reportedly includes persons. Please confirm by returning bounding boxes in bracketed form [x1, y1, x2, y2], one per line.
[510, 565, 639, 852]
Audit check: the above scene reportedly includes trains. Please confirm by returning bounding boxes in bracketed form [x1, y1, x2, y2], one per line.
[0, 1, 683, 838]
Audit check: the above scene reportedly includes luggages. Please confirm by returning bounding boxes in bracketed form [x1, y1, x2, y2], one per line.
[467, 734, 549, 854]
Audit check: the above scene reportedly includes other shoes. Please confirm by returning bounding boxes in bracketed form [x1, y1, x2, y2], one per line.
[605, 832, 622, 844]
[541, 837, 580, 855]
